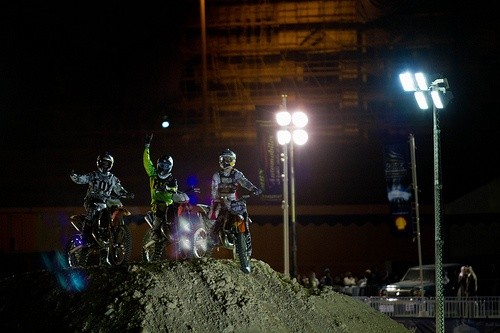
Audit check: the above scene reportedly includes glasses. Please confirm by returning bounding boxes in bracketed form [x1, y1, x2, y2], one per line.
[219, 156, 236, 164]
[97, 160, 111, 168]
[157, 163, 172, 170]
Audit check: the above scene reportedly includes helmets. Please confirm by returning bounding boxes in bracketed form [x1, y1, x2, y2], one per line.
[96, 153, 114, 173]
[219, 149, 236, 175]
[157, 155, 174, 179]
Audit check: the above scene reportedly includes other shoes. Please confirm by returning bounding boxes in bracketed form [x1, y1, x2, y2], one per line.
[209, 227, 220, 243]
[150, 247, 163, 264]
[77, 252, 88, 270]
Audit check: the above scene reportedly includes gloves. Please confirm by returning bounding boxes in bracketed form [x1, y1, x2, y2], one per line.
[251, 187, 262, 196]
[145, 133, 153, 145]
[126, 192, 135, 200]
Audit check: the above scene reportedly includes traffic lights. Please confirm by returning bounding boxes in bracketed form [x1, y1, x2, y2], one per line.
[392, 212, 411, 236]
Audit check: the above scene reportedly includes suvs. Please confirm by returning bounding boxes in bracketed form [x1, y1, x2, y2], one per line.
[380, 263, 480, 314]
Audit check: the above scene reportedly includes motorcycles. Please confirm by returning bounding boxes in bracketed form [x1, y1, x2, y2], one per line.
[64, 190, 132, 268]
[195, 193, 255, 273]
[140, 188, 214, 268]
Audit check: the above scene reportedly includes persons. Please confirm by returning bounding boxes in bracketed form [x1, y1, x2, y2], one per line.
[142, 130, 178, 264]
[69, 152, 128, 269]
[207, 148, 262, 259]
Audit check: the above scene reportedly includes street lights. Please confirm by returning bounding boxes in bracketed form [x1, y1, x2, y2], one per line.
[274, 94, 309, 284]
[398, 69, 453, 333]
